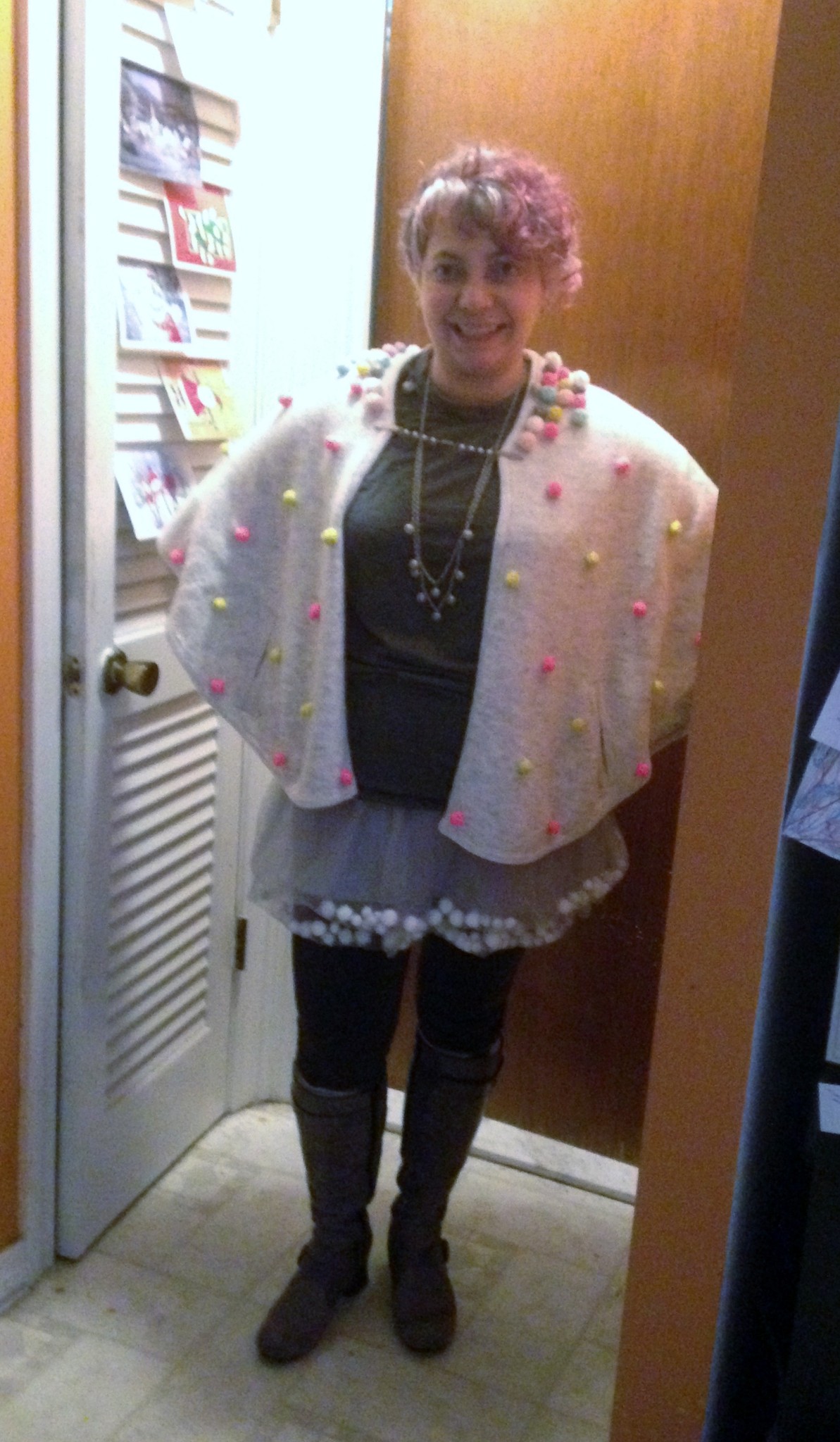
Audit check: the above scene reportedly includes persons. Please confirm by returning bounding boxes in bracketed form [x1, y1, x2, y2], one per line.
[157, 146, 719, 1368]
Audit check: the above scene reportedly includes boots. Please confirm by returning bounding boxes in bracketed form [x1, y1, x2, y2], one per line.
[257, 1068, 393, 1358]
[388, 1031, 508, 1357]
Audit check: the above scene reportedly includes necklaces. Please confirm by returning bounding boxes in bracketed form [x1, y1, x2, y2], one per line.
[407, 367, 524, 619]
